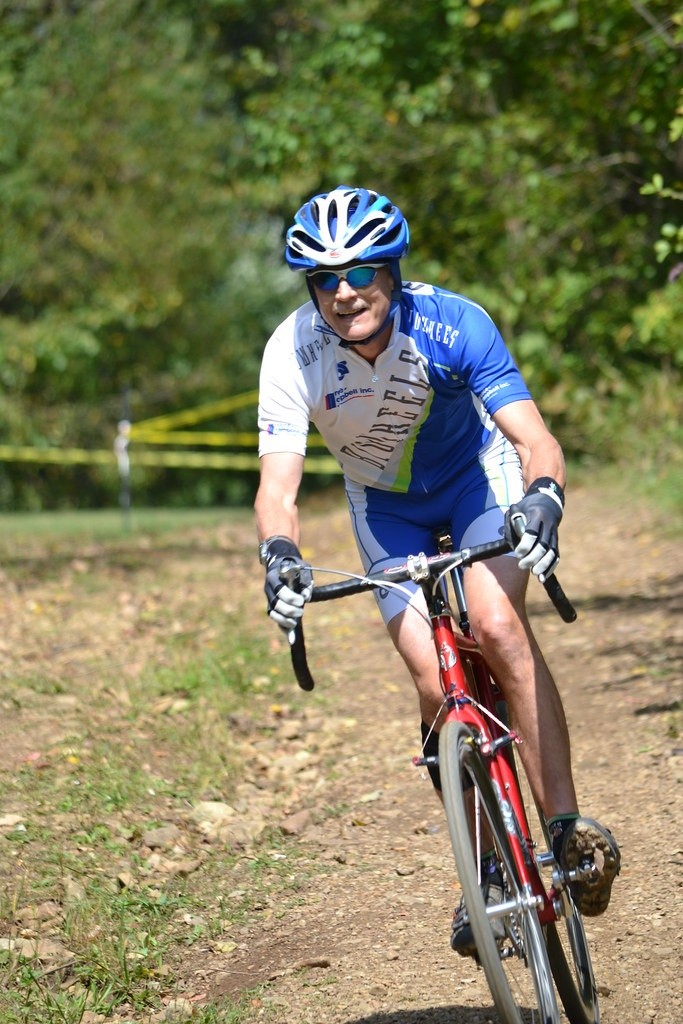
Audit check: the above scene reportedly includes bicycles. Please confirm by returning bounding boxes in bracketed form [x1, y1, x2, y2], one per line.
[277, 504, 606, 1023]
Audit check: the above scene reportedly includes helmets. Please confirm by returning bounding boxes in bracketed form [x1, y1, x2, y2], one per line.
[285, 184, 411, 273]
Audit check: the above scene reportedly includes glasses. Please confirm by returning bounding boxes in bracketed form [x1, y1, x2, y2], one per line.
[310, 266, 379, 291]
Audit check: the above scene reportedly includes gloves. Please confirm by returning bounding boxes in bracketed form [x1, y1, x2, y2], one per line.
[503, 477, 565, 582]
[258, 535, 314, 646]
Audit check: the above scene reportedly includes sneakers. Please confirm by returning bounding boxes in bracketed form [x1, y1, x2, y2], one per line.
[449, 865, 507, 955]
[553, 816, 620, 917]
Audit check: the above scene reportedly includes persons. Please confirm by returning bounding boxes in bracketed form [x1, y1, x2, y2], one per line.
[253, 186, 621, 966]
